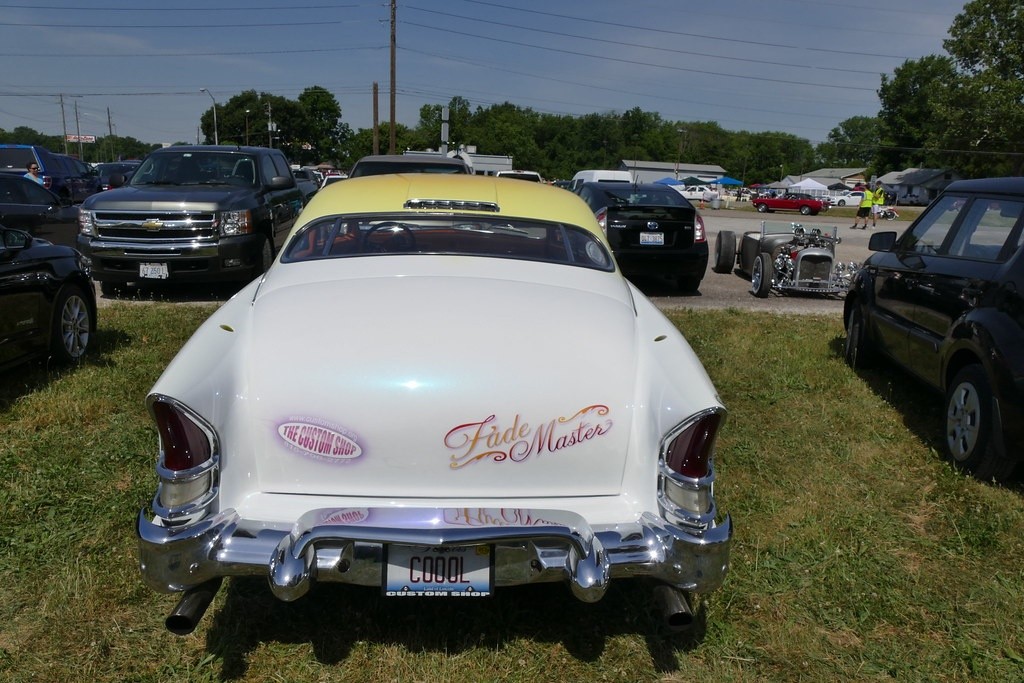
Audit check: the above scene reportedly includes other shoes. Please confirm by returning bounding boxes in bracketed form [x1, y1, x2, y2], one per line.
[850, 226, 857, 229]
[872, 226, 875, 228]
[862, 226, 867, 229]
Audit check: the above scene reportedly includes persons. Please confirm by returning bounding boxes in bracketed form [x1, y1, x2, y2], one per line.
[849, 180, 884, 229]
[771, 191, 778, 197]
[23, 162, 44, 187]
[739, 188, 751, 194]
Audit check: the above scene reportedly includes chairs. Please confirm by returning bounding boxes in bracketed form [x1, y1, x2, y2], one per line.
[236, 159, 256, 179]
[171, 157, 200, 178]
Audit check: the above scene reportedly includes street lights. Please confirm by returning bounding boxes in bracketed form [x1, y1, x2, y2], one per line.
[245, 109, 249, 145]
[200, 87, 218, 145]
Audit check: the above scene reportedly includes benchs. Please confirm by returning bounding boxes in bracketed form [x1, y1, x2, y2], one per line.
[332, 232, 549, 260]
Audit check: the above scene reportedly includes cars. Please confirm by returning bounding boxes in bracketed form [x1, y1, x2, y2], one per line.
[827, 191, 865, 207]
[574, 182, 709, 294]
[92, 163, 140, 192]
[679, 186, 717, 201]
[716, 219, 862, 300]
[0, 224, 97, 370]
[292, 168, 348, 201]
[897, 193, 921, 205]
[134, 173, 732, 638]
[842, 176, 1024, 472]
[752, 193, 828, 216]
[0, 173, 81, 245]
[552, 181, 571, 190]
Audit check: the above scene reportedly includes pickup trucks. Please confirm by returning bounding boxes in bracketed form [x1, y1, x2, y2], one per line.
[1, 144, 102, 206]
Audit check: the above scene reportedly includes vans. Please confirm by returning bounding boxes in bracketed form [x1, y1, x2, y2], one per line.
[567, 170, 634, 205]
[346, 155, 483, 233]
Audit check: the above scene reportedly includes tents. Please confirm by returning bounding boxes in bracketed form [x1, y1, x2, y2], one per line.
[748, 178, 828, 189]
[653, 176, 742, 186]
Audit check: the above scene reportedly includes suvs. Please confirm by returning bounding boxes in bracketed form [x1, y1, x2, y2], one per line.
[496, 170, 543, 184]
[77, 145, 305, 295]
